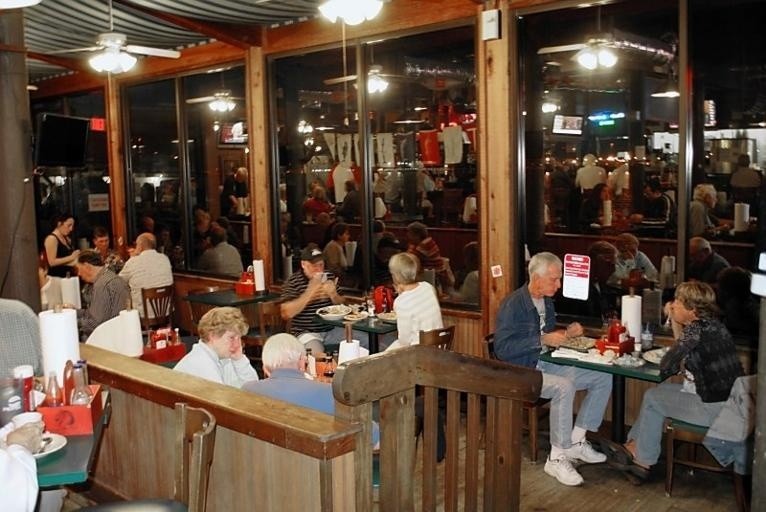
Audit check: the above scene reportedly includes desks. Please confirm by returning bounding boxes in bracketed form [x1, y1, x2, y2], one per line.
[234, 164, 628, 237]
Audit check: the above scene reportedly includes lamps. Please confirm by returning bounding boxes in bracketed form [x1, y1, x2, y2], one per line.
[568, 42, 621, 70]
[646, 64, 683, 101]
[317, 0, 385, 27]
[86, 51, 136, 75]
[205, 99, 239, 112]
[348, 74, 391, 95]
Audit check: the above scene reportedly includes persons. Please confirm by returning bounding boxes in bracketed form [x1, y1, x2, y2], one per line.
[382, 253, 446, 353]
[238, 331, 383, 449]
[490, 250, 608, 489]
[170, 304, 260, 391]
[43, 115, 765, 337]
[278, 241, 349, 362]
[0, 419, 187, 512]
[600, 279, 748, 486]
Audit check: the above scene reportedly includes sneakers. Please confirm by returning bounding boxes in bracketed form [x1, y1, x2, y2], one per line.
[598, 437, 652, 486]
[544, 437, 608, 486]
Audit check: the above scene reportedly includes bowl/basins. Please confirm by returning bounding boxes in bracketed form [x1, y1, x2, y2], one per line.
[315, 305, 352, 321]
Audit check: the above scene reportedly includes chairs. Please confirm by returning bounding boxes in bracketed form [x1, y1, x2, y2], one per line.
[41, 246, 453, 496]
[660, 367, 759, 511]
[477, 333, 550, 462]
[79, 398, 217, 512]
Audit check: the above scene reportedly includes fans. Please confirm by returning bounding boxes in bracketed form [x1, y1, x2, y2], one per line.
[322, 43, 415, 86]
[41, 1, 182, 60]
[536, 4, 636, 55]
[183, 69, 248, 104]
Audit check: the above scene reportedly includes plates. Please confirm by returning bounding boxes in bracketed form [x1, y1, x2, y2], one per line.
[559, 336, 598, 351]
[343, 310, 368, 321]
[642, 348, 663, 364]
[613, 356, 645, 369]
[0, 434, 68, 459]
[375, 312, 395, 321]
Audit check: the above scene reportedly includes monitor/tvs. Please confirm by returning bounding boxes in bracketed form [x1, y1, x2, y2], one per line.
[668, 99, 717, 131]
[584, 104, 629, 139]
[217, 117, 249, 149]
[551, 113, 584, 136]
[34, 112, 90, 175]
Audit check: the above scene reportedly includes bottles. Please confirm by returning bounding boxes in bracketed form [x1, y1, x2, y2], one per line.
[600, 317, 630, 344]
[322, 353, 334, 383]
[640, 323, 653, 353]
[12, 364, 35, 412]
[63, 358, 91, 407]
[304, 347, 314, 372]
[45, 372, 62, 407]
[365, 286, 394, 314]
[150, 326, 181, 349]
[624, 265, 646, 280]
[366, 305, 375, 318]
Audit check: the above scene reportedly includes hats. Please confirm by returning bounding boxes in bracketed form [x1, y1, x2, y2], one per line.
[300, 245, 329, 265]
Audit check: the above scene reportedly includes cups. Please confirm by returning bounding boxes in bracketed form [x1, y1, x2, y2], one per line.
[11, 411, 41, 430]
